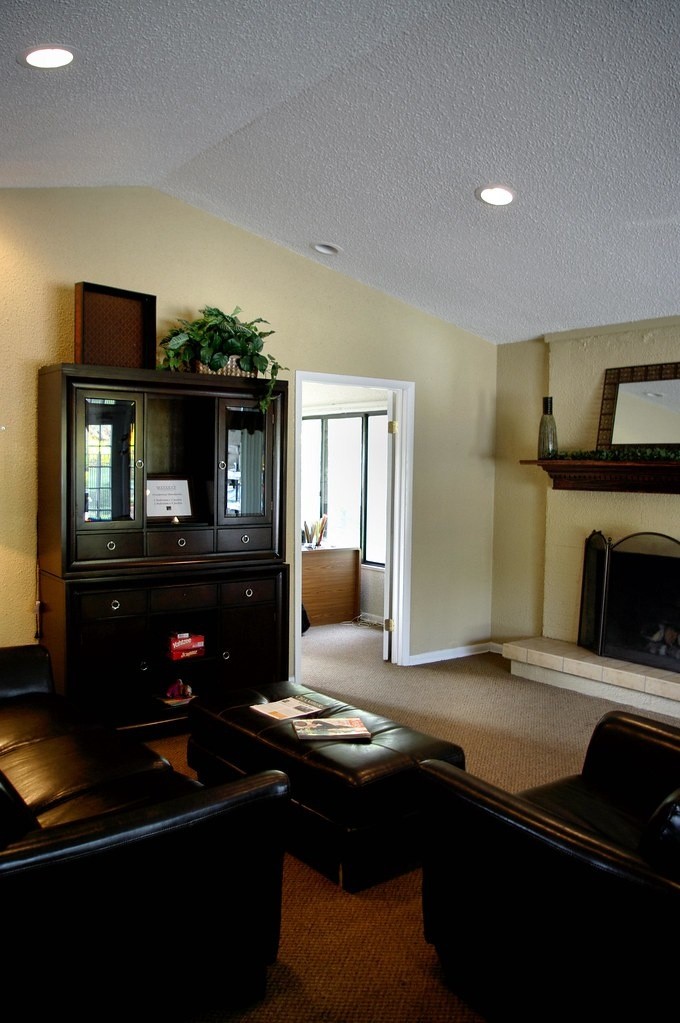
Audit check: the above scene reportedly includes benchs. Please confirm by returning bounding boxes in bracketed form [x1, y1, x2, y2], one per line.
[186, 680, 466, 895]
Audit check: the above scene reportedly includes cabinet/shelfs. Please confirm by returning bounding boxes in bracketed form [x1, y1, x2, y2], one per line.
[36, 363, 290, 744]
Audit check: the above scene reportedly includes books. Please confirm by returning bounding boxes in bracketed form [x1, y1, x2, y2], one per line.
[292, 717, 372, 739]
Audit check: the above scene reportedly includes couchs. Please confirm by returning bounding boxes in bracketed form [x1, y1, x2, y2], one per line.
[0, 643, 291, 1023]
[417, 709, 680, 1023]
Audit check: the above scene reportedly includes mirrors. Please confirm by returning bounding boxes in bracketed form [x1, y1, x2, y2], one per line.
[596, 362, 680, 451]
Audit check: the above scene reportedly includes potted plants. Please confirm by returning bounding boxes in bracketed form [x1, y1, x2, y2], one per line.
[159, 306, 291, 414]
